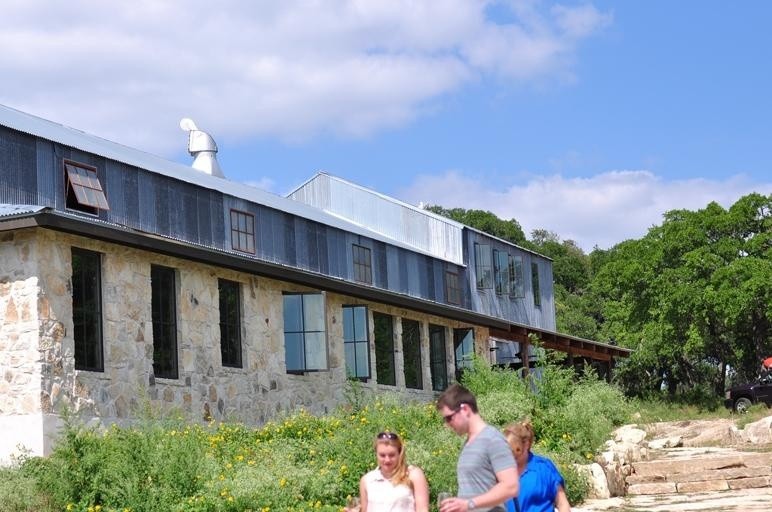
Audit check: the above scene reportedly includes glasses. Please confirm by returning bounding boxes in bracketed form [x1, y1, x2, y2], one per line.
[445, 410, 460, 423]
[377, 433, 396, 439]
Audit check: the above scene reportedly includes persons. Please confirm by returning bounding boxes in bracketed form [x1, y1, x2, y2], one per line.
[502, 420, 572, 512]
[343, 433, 430, 512]
[436, 382, 520, 512]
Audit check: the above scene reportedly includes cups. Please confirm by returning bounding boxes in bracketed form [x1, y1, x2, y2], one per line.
[345, 496, 361, 512]
[437, 492, 453, 511]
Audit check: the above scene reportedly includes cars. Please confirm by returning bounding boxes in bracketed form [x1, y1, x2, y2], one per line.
[723, 370, 772, 414]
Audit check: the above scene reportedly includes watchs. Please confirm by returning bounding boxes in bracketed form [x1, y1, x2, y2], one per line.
[468, 498, 477, 511]
